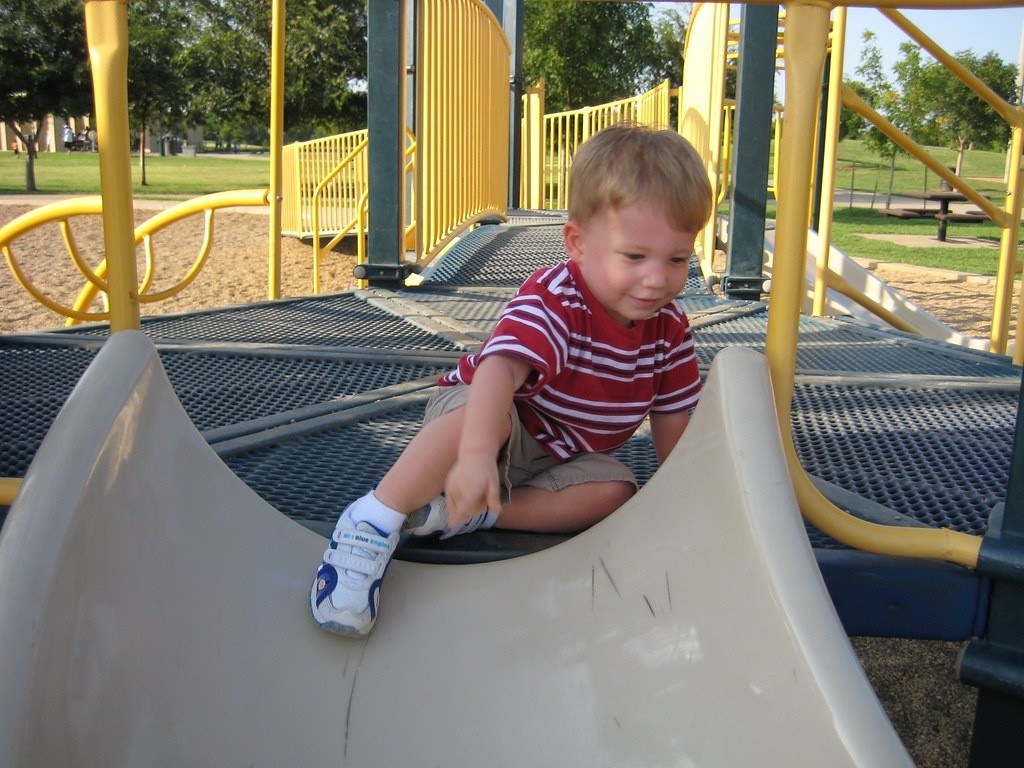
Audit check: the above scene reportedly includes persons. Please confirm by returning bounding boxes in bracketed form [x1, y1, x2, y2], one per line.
[215, 131, 241, 154]
[310, 118, 712, 637]
[12, 124, 97, 158]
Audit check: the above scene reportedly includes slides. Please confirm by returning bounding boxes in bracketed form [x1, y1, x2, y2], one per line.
[716, 212, 1015, 360]
[0, 330, 923, 768]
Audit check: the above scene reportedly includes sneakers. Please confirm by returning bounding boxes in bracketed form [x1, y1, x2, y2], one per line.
[310, 501, 400, 638]
[401, 491, 489, 539]
